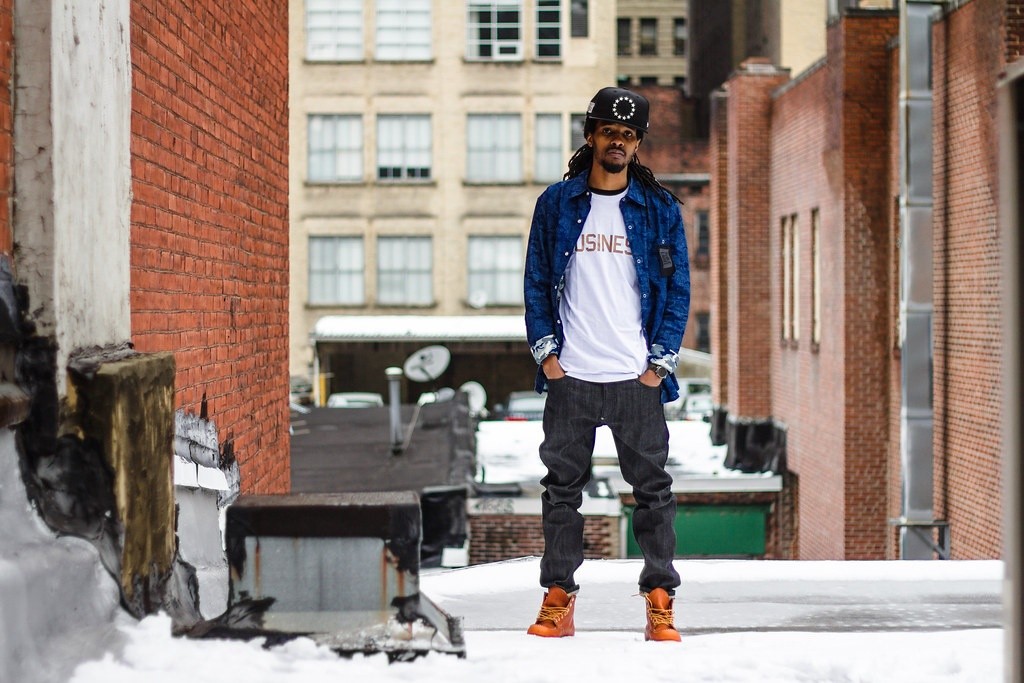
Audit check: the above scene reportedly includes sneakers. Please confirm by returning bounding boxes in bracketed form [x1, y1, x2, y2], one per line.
[641, 588, 681, 642]
[526, 586, 577, 638]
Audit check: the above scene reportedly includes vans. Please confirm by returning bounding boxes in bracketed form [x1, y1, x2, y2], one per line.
[659, 379, 715, 422]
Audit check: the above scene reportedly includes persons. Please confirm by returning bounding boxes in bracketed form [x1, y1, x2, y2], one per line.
[523, 86, 692, 642]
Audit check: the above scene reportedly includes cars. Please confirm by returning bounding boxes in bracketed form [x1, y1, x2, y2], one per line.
[327, 391, 383, 408]
[493, 391, 549, 421]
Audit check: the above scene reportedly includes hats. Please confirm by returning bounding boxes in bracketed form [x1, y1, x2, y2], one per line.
[585, 87, 650, 133]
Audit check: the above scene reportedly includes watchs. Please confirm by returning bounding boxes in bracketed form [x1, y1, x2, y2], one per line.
[647, 364, 668, 379]
